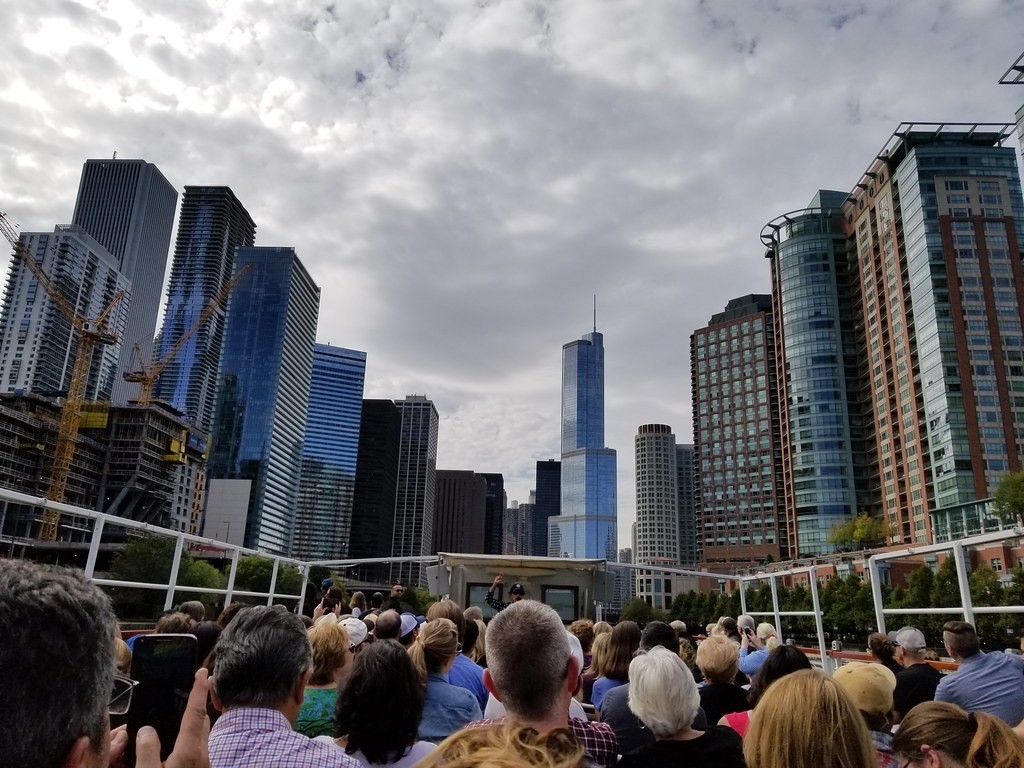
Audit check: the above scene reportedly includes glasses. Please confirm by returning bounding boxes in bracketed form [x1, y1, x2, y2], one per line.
[393, 589, 403, 593]
[413, 624, 420, 630]
[511, 592, 524, 595]
[104, 674, 140, 729]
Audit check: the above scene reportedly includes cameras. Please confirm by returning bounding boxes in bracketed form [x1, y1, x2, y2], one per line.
[745, 628, 750, 637]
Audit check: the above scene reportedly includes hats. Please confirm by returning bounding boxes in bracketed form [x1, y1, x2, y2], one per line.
[510, 583, 525, 593]
[338, 617, 367, 647]
[565, 629, 585, 676]
[398, 612, 426, 637]
[363, 613, 378, 635]
[322, 578, 333, 590]
[887, 627, 926, 653]
[886, 631, 898, 645]
[832, 661, 896, 715]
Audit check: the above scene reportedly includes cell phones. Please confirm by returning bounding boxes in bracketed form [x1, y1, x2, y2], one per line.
[121, 634, 199, 768]
[323, 598, 339, 608]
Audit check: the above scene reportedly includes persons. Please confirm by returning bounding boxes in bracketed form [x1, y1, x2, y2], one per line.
[0, 585, 1024, 768]
[485, 571, 525, 612]
